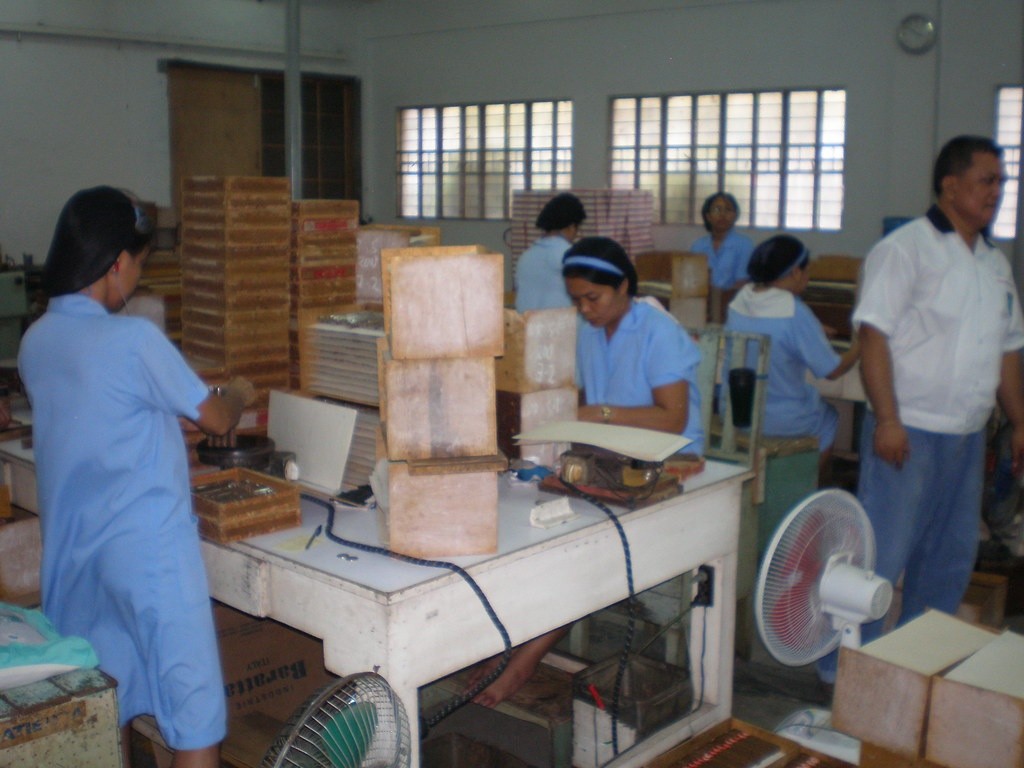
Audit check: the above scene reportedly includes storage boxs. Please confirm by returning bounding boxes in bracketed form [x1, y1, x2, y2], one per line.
[643, 717, 857, 768]
[133, 177, 708, 562]
[151, 599, 324, 767]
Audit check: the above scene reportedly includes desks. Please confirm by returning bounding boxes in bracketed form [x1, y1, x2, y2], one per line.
[0, 429, 761, 768]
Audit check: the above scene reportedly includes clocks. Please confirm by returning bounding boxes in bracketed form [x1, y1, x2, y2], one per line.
[892, 14, 939, 55]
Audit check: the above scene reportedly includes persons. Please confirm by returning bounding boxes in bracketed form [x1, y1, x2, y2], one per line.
[468, 236, 700, 710]
[17, 187, 258, 768]
[689, 193, 753, 318]
[716, 235, 862, 474]
[512, 193, 586, 314]
[818, 134, 1024, 711]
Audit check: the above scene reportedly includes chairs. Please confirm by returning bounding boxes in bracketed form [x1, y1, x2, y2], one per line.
[569, 329, 772, 667]
[713, 330, 827, 559]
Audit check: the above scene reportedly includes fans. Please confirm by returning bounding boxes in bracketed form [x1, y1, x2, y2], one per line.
[259, 671, 413, 767]
[753, 487, 896, 764]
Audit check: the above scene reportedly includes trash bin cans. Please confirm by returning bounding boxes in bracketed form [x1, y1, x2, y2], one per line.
[570, 649, 692, 768]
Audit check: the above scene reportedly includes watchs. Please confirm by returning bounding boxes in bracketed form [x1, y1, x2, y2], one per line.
[601, 405, 610, 423]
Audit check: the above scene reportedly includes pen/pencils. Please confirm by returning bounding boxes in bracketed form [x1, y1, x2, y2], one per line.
[305, 525, 322, 549]
